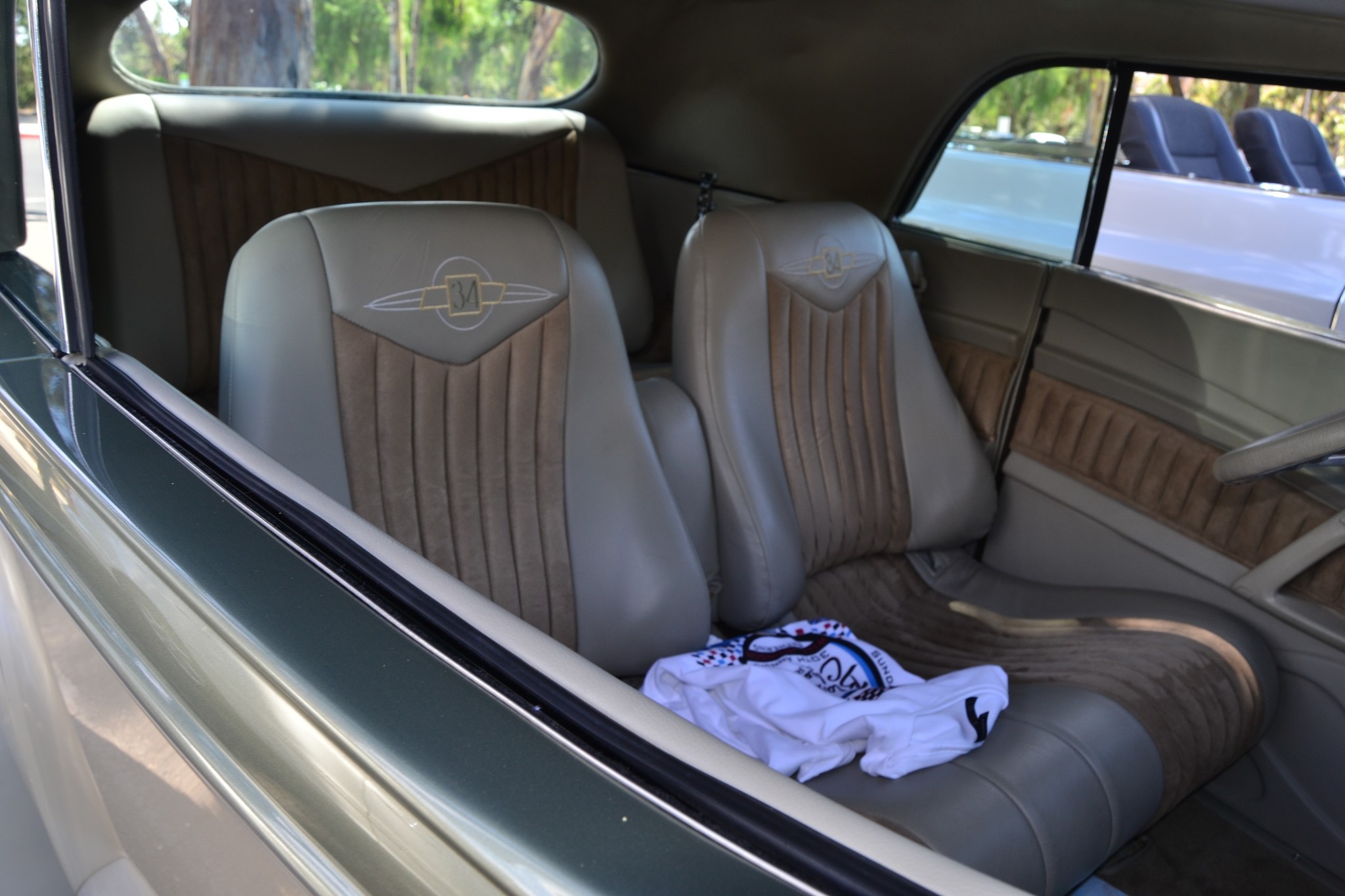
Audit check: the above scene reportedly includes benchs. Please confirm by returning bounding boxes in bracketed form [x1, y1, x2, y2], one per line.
[70, 90, 658, 426]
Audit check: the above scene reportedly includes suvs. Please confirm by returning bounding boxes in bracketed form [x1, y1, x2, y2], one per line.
[0, 1, 1345, 896]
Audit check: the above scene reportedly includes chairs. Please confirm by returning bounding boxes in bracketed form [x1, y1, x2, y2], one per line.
[1233, 107, 1345, 197]
[223, 201, 1282, 896]
[1119, 96, 1255, 184]
